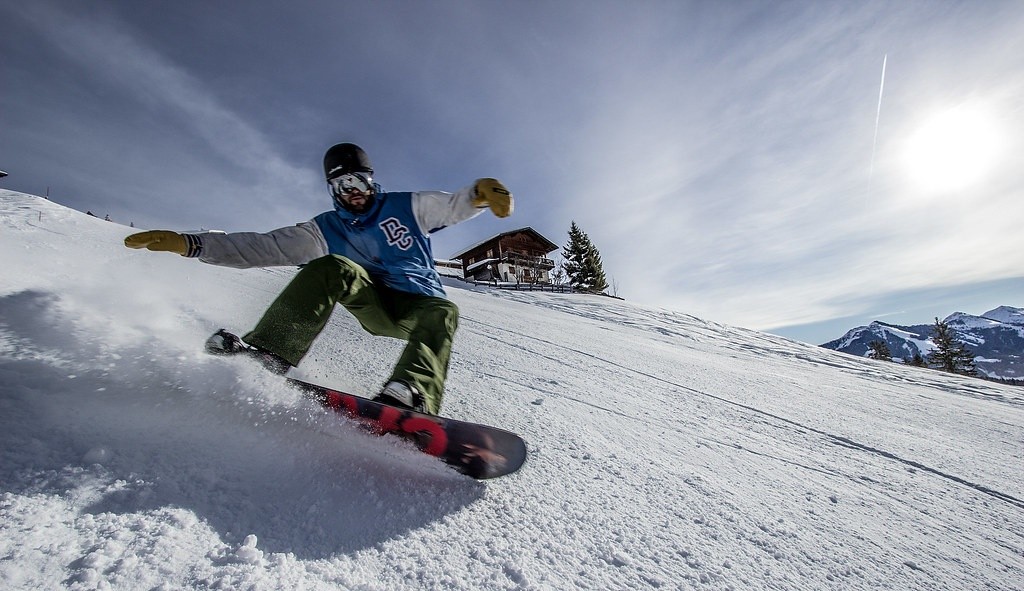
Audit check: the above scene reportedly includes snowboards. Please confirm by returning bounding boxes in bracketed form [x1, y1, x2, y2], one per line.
[206, 348, 526, 480]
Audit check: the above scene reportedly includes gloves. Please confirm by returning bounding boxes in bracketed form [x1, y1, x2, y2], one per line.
[471, 178, 514, 218]
[125, 230, 187, 255]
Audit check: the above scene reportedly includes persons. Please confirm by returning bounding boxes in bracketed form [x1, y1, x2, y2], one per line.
[123, 140, 515, 417]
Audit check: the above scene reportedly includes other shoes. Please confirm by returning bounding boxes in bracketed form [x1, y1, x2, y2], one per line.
[380, 381, 427, 414]
[206, 329, 291, 375]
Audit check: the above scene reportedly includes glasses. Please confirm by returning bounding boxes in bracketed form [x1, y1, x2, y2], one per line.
[329, 172, 372, 195]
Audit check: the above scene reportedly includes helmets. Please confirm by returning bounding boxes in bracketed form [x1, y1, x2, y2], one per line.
[324, 143, 374, 183]
